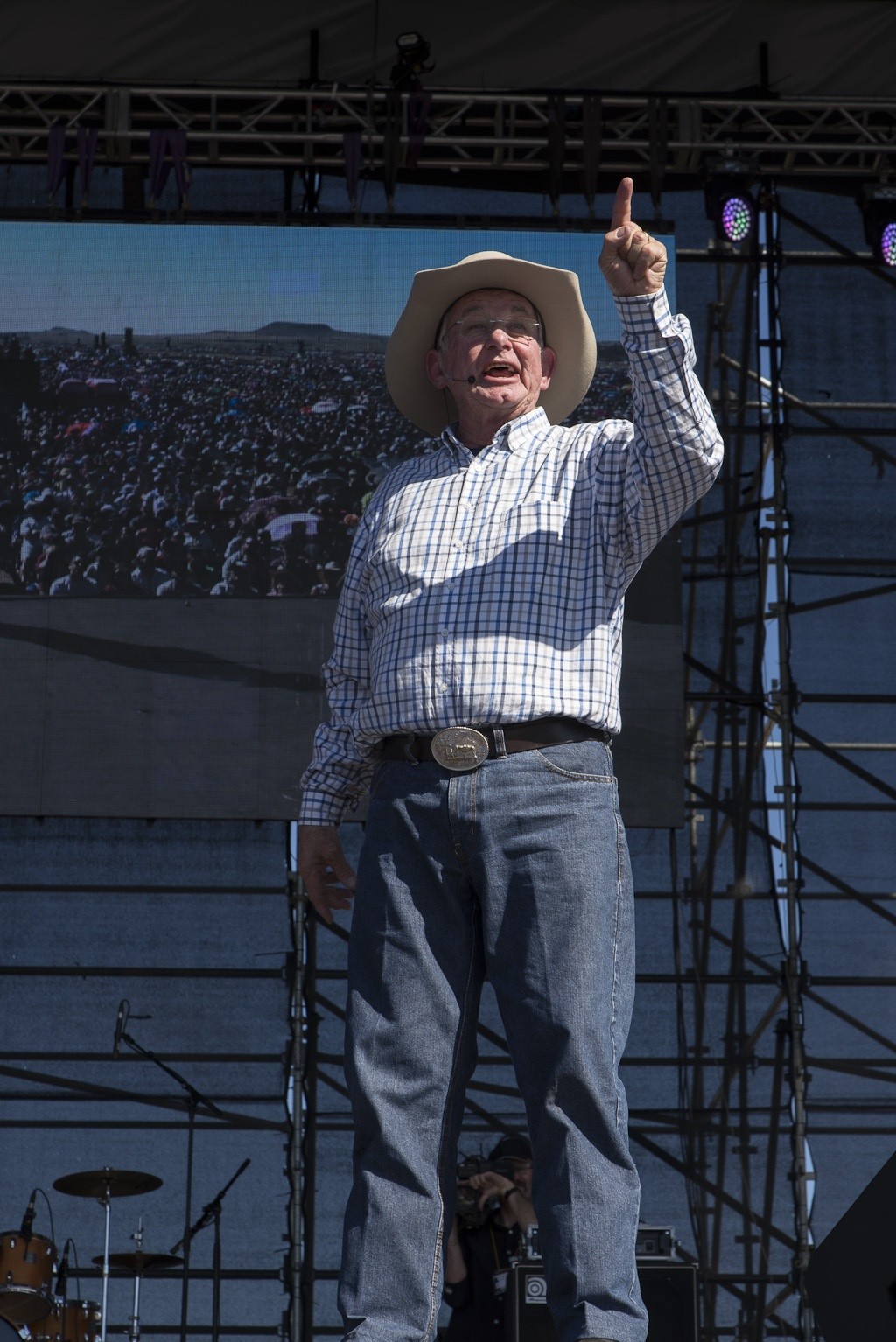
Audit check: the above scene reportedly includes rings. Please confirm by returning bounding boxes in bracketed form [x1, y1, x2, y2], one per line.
[643, 231, 649, 241]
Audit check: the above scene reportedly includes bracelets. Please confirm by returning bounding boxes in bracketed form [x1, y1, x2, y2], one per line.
[503, 1187, 517, 1202]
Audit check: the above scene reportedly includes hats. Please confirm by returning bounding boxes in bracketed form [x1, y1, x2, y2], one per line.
[384, 251, 597, 433]
[489, 1138, 533, 1162]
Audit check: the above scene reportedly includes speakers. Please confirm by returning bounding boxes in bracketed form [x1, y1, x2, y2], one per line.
[511, 1262, 703, 1342]
[805, 1148, 896, 1342]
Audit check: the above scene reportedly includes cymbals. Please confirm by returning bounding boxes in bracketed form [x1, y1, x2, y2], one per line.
[52, 1170, 163, 1198]
[91, 1250, 184, 1271]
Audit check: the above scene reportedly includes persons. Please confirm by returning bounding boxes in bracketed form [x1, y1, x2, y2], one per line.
[297, 176, 725, 1342]
[442, 1132, 538, 1342]
[0, 348, 436, 603]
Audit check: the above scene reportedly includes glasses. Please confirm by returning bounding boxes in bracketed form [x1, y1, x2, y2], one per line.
[438, 317, 546, 346]
[513, 1164, 531, 1171]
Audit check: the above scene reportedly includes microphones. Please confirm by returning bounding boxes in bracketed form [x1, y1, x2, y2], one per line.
[112, 1002, 123, 1059]
[18, 1190, 36, 1242]
[55, 1243, 70, 1296]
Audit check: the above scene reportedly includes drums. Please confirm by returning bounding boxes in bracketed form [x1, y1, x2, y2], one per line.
[0, 1312, 27, 1342]
[26, 1299, 101, 1342]
[0, 1231, 58, 1324]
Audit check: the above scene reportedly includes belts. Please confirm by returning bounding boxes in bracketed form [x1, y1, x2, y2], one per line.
[367, 715, 605, 771]
